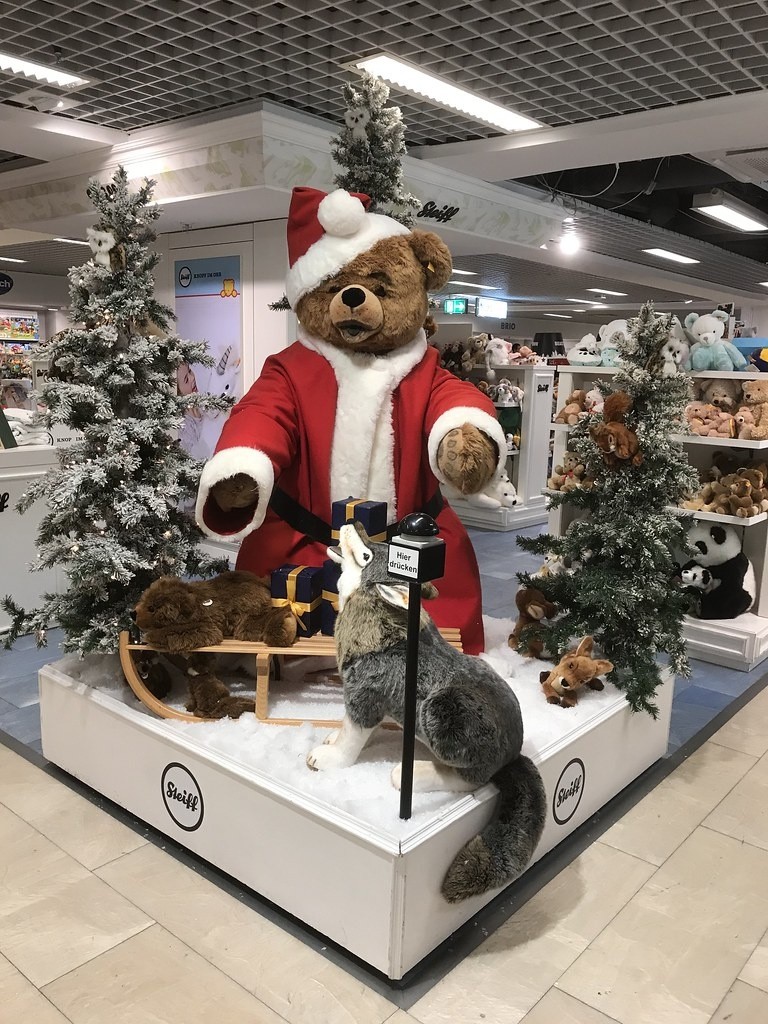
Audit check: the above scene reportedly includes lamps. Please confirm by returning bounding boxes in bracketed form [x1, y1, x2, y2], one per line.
[689, 189, 768, 234]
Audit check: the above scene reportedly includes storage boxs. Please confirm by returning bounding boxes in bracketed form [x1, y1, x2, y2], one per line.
[330, 496, 388, 547]
[321, 559, 342, 637]
[270, 563, 320, 637]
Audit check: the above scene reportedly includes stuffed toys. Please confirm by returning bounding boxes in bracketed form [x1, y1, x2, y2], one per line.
[426, 333, 559, 510]
[130, 187, 645, 904]
[547, 310, 768, 620]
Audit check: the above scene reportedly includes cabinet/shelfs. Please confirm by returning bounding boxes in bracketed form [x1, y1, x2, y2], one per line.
[438, 333, 556, 533]
[518, 312, 768, 673]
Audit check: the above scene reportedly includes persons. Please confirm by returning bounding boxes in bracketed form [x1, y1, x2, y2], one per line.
[176, 361, 204, 453]
